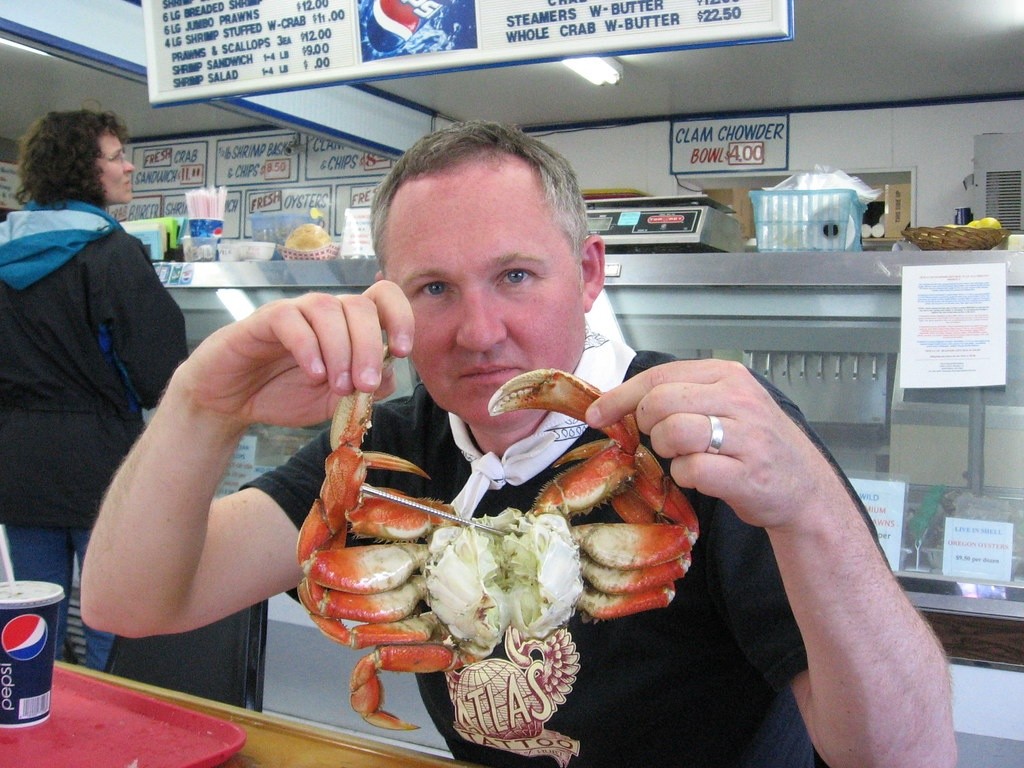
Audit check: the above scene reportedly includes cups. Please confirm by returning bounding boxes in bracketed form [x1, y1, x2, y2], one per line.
[0, 580, 65, 728]
[187, 219, 224, 259]
[955, 207, 971, 225]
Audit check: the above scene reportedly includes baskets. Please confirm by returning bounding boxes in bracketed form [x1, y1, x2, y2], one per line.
[899, 225, 1012, 251]
[277, 242, 340, 259]
[747, 188, 868, 252]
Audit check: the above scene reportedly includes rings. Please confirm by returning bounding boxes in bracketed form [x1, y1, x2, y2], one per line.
[707, 416, 724, 455]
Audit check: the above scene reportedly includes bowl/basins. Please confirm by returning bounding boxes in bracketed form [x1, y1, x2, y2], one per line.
[180, 236, 216, 263]
[218, 243, 245, 261]
[239, 240, 275, 261]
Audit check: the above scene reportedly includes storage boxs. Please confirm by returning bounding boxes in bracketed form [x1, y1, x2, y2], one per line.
[704, 184, 911, 251]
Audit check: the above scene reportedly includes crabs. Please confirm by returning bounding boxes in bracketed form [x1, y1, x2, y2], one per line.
[294, 341, 702, 732]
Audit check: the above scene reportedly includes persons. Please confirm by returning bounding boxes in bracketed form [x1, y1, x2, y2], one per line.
[81, 120, 958, 768]
[0, 110, 190, 672]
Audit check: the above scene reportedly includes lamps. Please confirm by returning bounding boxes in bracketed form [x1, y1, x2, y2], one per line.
[562, 56, 624, 87]
